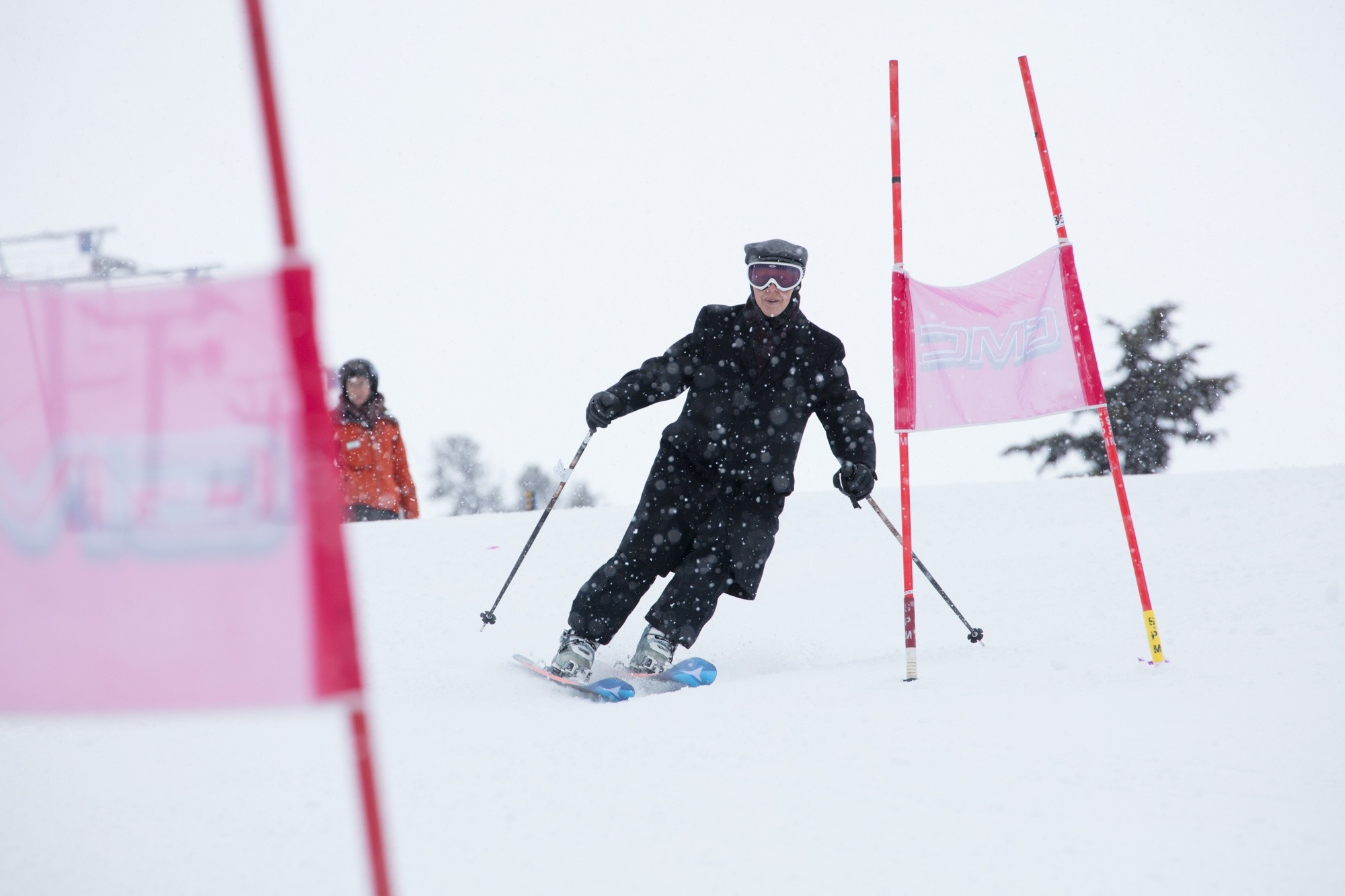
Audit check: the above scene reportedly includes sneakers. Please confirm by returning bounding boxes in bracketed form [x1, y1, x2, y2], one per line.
[548, 630, 599, 684]
[629, 623, 679, 676]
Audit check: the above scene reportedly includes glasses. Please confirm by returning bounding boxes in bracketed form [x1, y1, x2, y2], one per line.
[747, 262, 804, 291]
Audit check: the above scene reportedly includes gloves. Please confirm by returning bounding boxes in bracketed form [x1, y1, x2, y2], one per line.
[833, 463, 875, 501]
[586, 390, 624, 432]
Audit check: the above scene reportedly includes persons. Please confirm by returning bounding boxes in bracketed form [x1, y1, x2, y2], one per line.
[330, 358, 419, 522]
[551, 239, 876, 680]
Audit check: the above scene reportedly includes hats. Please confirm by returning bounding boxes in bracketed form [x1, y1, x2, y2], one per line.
[338, 358, 378, 397]
[744, 238, 808, 271]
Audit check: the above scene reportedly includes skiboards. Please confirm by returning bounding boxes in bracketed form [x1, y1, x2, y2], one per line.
[509, 651, 719, 702]
